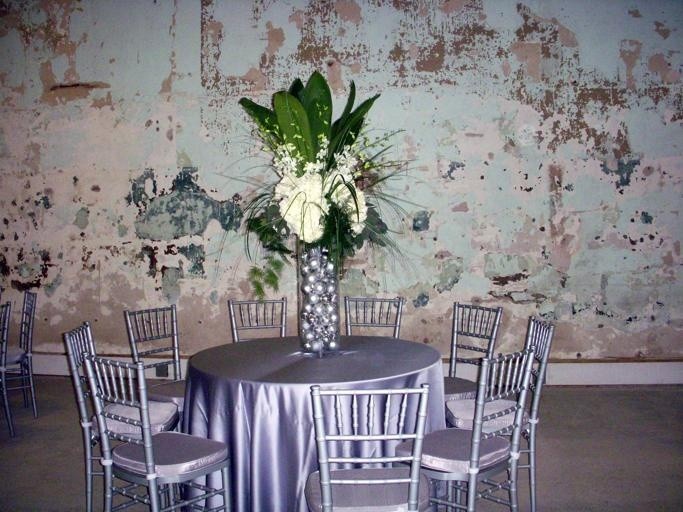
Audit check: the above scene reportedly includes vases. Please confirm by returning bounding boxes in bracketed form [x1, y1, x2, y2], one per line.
[294, 234, 341, 353]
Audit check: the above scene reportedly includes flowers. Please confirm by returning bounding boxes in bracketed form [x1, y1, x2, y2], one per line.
[212, 69, 433, 283]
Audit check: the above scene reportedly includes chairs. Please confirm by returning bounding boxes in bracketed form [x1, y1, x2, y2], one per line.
[81, 351, 230, 512]
[61, 321, 179, 512]
[1, 301, 15, 438]
[445, 315, 555, 512]
[443, 302, 502, 404]
[125, 304, 187, 431]
[395, 344, 535, 512]
[228, 297, 287, 343]
[304, 383, 437, 512]
[345, 296, 404, 338]
[1, 290, 38, 425]
